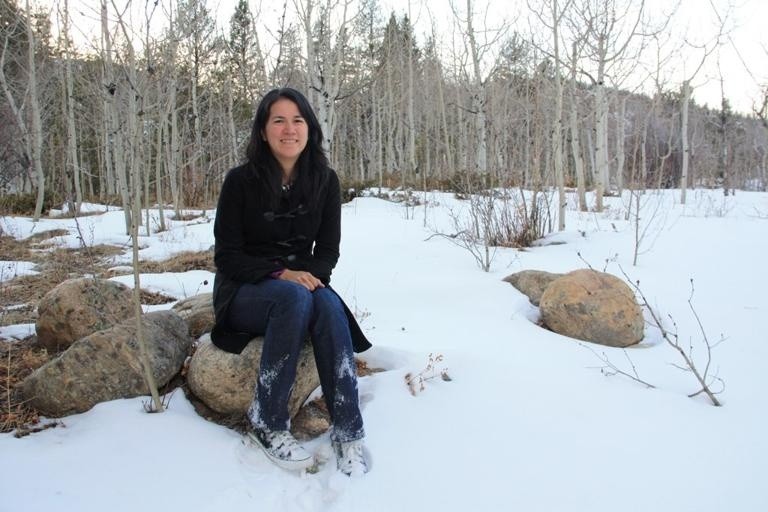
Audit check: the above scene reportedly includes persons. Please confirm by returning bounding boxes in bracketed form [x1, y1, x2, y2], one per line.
[209, 89, 371, 475]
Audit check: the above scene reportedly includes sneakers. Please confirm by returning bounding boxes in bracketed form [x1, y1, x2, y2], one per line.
[329, 425, 367, 474]
[247, 426, 314, 470]
